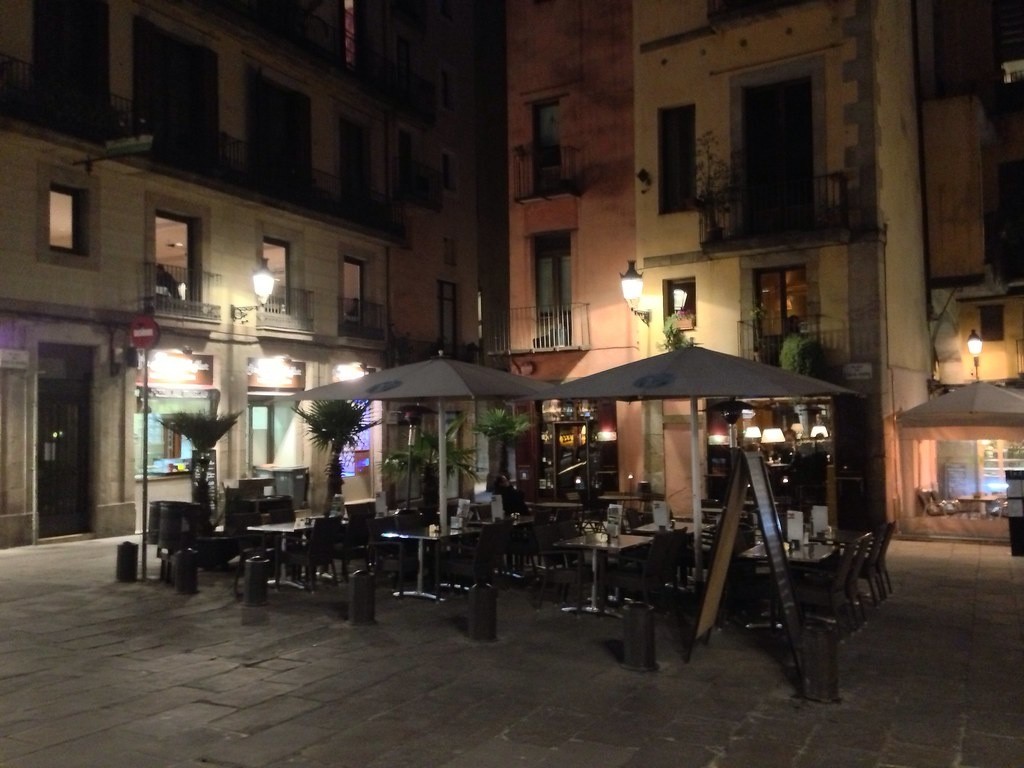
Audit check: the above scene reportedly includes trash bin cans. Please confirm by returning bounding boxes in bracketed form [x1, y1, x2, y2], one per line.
[146, 501, 159, 545]
[156, 501, 201, 558]
[253, 463, 310, 510]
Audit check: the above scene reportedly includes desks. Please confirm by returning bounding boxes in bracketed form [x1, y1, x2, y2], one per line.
[380, 526, 483, 603]
[535, 502, 584, 547]
[957, 496, 998, 514]
[734, 541, 840, 632]
[245, 522, 316, 591]
[467, 515, 535, 579]
[552, 531, 655, 619]
[631, 520, 716, 591]
[597, 495, 645, 534]
[810, 530, 873, 546]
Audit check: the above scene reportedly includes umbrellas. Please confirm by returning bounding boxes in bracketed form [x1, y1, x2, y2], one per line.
[893, 382, 1024, 496]
[512, 336, 863, 598]
[262, 349, 575, 536]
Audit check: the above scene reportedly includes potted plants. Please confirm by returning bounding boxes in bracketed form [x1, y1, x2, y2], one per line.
[692, 130, 734, 243]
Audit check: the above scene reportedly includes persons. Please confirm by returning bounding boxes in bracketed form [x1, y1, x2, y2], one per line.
[154, 263, 180, 308]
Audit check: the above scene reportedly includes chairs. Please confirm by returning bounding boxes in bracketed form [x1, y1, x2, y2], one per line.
[150, 478, 897, 663]
[177, 282, 187, 301]
[917, 490, 1011, 519]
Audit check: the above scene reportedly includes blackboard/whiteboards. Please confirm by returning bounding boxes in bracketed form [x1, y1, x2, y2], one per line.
[192, 450, 217, 517]
[693, 447, 803, 649]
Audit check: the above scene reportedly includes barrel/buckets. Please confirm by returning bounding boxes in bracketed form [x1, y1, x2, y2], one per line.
[147, 501, 200, 557]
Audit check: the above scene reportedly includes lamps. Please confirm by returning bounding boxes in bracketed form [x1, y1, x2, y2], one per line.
[126, 346, 139, 367]
[670, 284, 687, 312]
[965, 329, 982, 385]
[618, 260, 650, 329]
[694, 396, 829, 466]
[230, 256, 276, 324]
